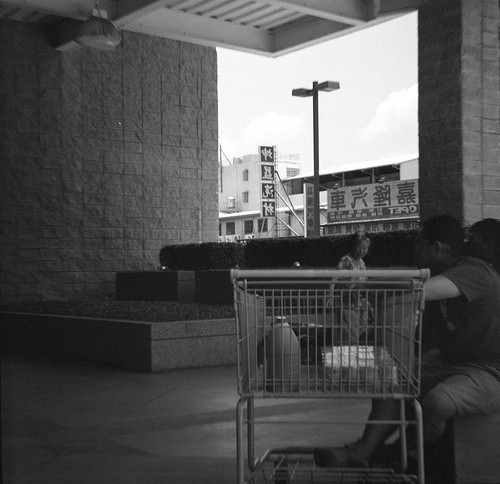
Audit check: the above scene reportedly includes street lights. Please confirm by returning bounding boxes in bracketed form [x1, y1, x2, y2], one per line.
[290, 80, 339, 235]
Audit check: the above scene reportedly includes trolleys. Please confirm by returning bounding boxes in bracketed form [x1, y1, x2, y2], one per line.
[235, 263, 429, 484]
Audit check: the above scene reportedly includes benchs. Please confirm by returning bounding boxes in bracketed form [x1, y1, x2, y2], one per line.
[0, 311, 338, 374]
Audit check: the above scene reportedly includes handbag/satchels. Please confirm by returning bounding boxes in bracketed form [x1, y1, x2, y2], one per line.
[338, 295, 376, 330]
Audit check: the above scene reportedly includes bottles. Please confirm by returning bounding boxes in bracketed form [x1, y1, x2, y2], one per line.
[266, 316, 301, 393]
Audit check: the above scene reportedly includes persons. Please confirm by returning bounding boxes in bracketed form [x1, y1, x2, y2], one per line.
[312, 212, 499, 471]
[325, 230, 374, 343]
[463, 216, 500, 271]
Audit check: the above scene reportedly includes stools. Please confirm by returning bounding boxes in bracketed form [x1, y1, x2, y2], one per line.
[398, 409, 500, 484]
